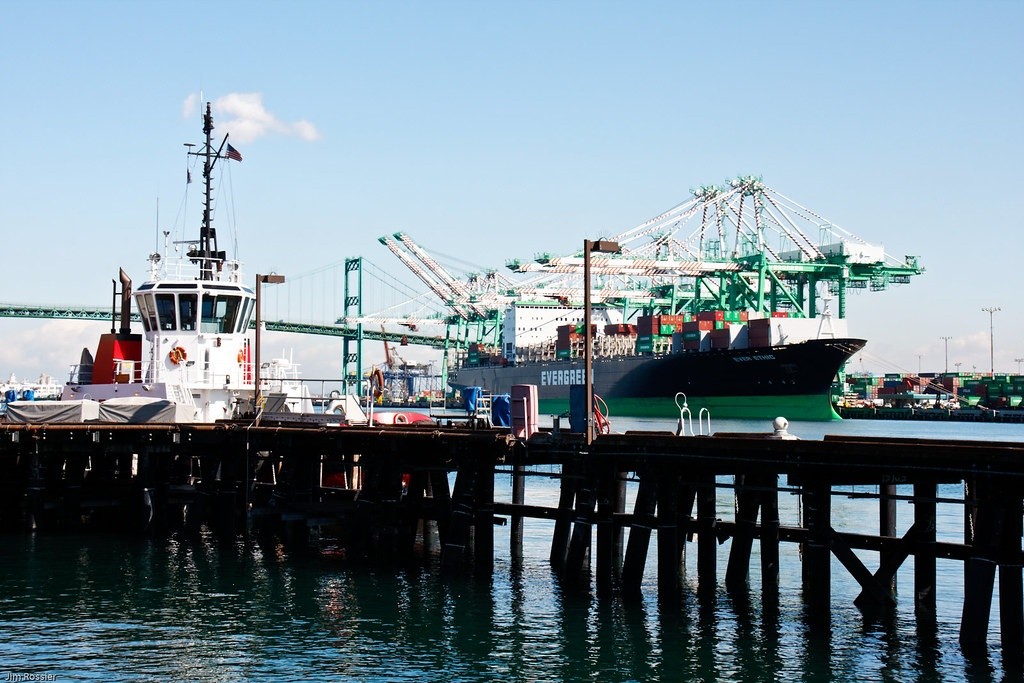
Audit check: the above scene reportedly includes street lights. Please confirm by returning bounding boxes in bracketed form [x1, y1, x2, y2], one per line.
[255, 273, 286, 423]
[580, 237, 619, 443]
[982, 307, 1002, 379]
[940, 336, 952, 373]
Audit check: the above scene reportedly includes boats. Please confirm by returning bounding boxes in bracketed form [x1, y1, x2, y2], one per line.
[61, 87, 316, 425]
[379, 174, 930, 426]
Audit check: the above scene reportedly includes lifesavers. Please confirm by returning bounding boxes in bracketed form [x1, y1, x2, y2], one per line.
[369, 367, 386, 401]
[167, 344, 190, 367]
